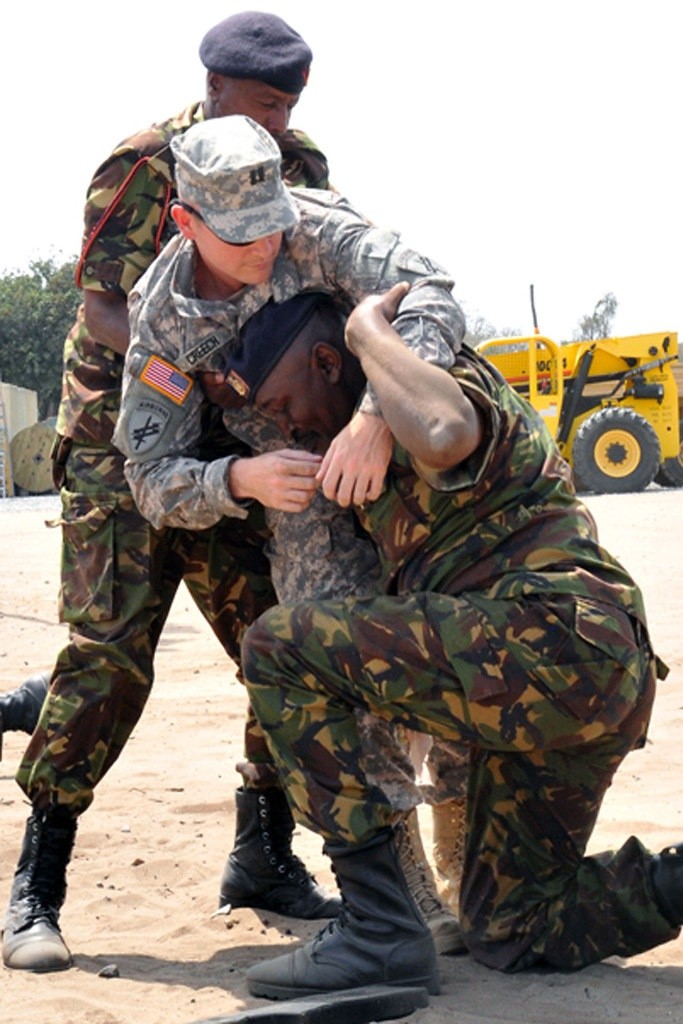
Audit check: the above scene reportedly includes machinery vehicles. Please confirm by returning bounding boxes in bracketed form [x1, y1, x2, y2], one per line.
[468, 285, 683, 492]
[648, 348, 683, 488]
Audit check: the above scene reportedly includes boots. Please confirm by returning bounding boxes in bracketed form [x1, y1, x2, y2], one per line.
[395, 795, 470, 955]
[1, 803, 79, 973]
[219, 786, 342, 920]
[0, 672, 52, 761]
[653, 841, 683, 926]
[248, 826, 440, 1001]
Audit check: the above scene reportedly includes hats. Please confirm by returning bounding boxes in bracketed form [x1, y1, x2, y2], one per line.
[170, 114, 300, 243]
[223, 289, 329, 405]
[200, 11, 312, 94]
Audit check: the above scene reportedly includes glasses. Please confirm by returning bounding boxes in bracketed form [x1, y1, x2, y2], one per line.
[167, 198, 256, 247]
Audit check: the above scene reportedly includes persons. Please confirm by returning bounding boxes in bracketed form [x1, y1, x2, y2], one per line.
[218, 285, 683, 999]
[0, 672, 54, 734]
[109, 113, 476, 957]
[0, 10, 348, 974]
